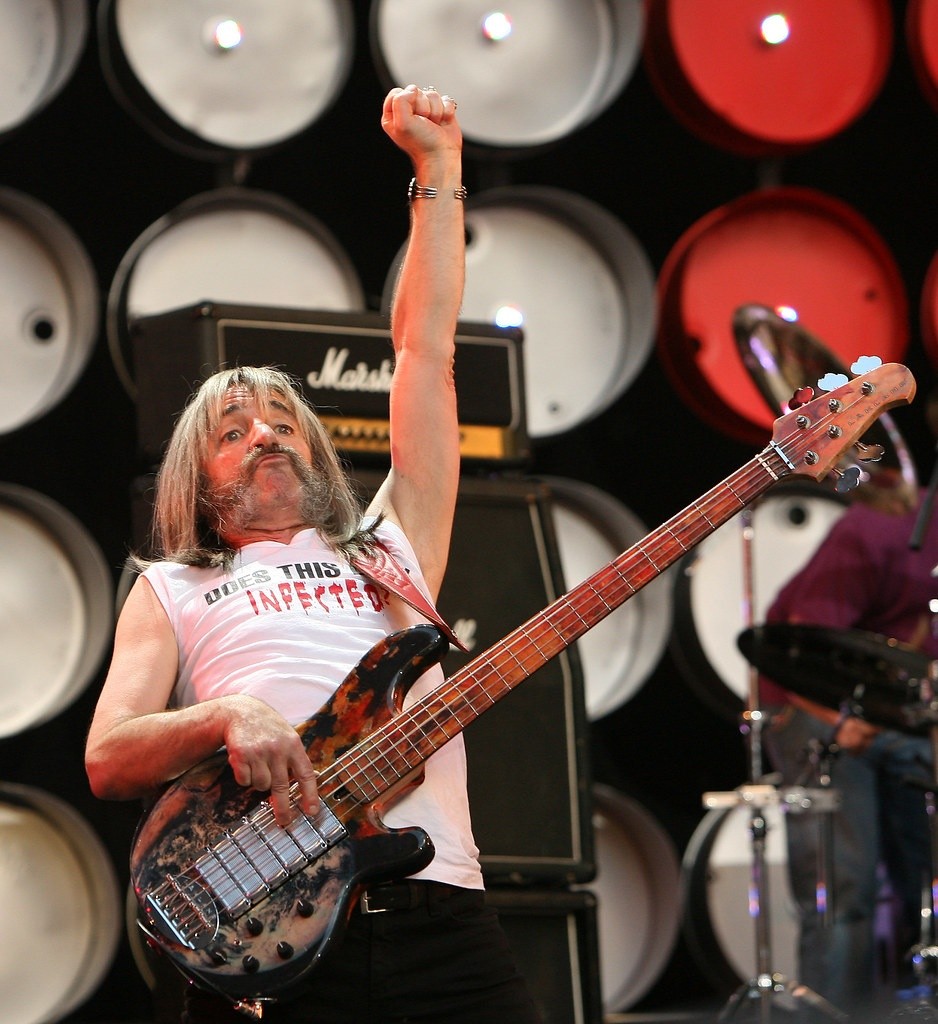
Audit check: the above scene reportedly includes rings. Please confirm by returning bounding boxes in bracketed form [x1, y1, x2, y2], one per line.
[443, 95, 457, 109]
[422, 86, 437, 91]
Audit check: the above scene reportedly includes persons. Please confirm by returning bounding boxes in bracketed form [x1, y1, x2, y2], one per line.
[755, 483, 938, 997]
[83, 85, 543, 1024]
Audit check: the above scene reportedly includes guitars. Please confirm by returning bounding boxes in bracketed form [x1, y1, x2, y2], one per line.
[127, 355, 919, 1018]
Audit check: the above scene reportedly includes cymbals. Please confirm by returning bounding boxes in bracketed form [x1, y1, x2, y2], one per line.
[737, 621, 938, 743]
[731, 305, 914, 499]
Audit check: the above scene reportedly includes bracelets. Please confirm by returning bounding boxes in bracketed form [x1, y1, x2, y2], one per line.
[408, 177, 467, 200]
